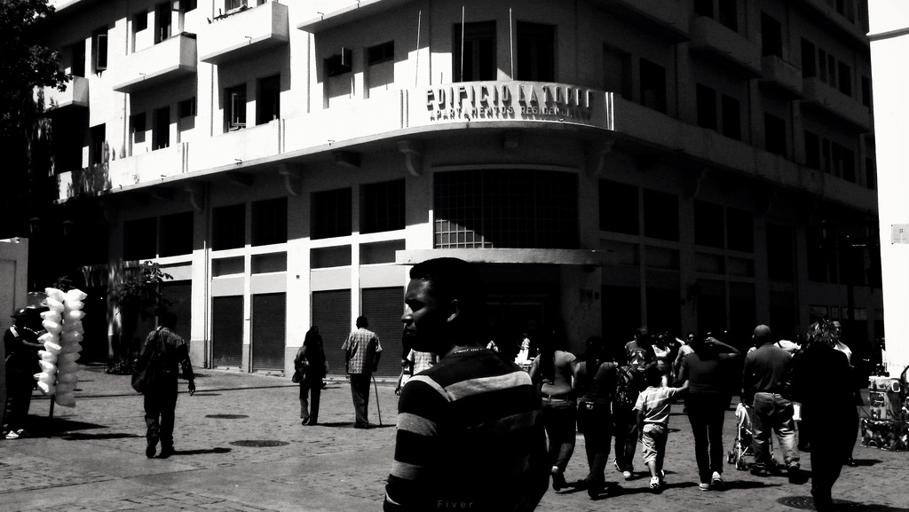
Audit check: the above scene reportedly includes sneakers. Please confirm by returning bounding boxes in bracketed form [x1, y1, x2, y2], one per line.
[300, 416, 318, 425]
[159, 448, 175, 459]
[846, 456, 858, 467]
[145, 434, 159, 459]
[698, 481, 711, 492]
[354, 420, 371, 428]
[712, 471, 722, 483]
[789, 467, 802, 484]
[0, 427, 29, 440]
[751, 467, 770, 478]
[548, 460, 668, 500]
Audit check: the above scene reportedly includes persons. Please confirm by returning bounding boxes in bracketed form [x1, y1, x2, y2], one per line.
[379, 256, 560, 512]
[395, 317, 442, 395]
[340, 316, 383, 429]
[0, 308, 44, 439]
[530, 317, 863, 502]
[311, 325, 326, 388]
[131, 312, 195, 461]
[291, 330, 326, 425]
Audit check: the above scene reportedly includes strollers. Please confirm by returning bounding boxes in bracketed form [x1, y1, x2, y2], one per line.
[727, 402, 778, 472]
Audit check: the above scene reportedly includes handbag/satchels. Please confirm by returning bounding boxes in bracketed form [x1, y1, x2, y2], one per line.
[131, 359, 171, 392]
